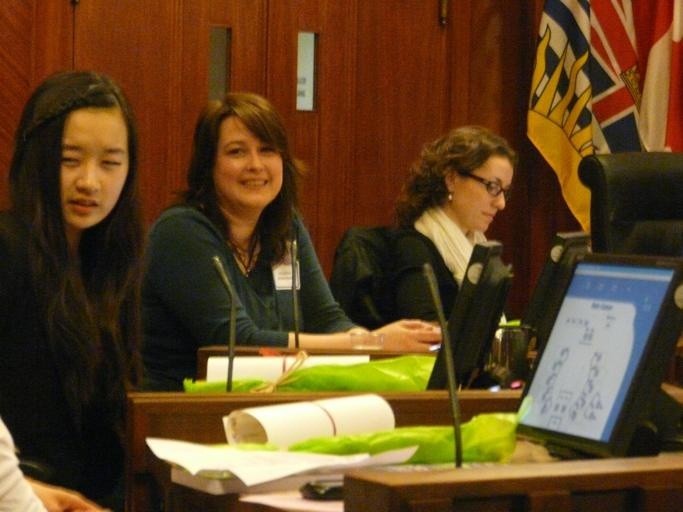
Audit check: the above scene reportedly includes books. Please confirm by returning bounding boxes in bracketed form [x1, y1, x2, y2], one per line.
[169, 466, 345, 498]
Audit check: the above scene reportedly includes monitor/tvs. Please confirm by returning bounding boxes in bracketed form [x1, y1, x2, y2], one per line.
[425, 241, 512, 389]
[512, 249, 683, 460]
[519, 231, 592, 342]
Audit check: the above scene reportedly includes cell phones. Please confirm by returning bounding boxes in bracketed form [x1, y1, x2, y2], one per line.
[299, 479, 343, 500]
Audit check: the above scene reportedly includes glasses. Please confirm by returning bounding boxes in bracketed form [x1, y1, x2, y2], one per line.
[457, 172, 512, 198]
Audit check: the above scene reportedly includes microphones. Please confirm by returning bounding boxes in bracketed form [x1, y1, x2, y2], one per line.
[422, 263, 464, 469]
[211, 254, 242, 391]
[289, 232, 301, 348]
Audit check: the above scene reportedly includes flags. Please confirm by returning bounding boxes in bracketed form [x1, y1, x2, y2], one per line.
[523, 0, 683, 248]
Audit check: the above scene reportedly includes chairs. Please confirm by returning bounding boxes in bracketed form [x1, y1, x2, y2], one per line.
[537, 151, 681, 255]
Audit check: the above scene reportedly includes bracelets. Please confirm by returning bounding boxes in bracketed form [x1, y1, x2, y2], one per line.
[350, 326, 367, 349]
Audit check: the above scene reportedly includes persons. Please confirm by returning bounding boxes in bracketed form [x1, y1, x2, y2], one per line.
[140, 92, 444, 391]
[370, 123, 522, 390]
[0, 414, 111, 511]
[0, 67, 167, 511]
